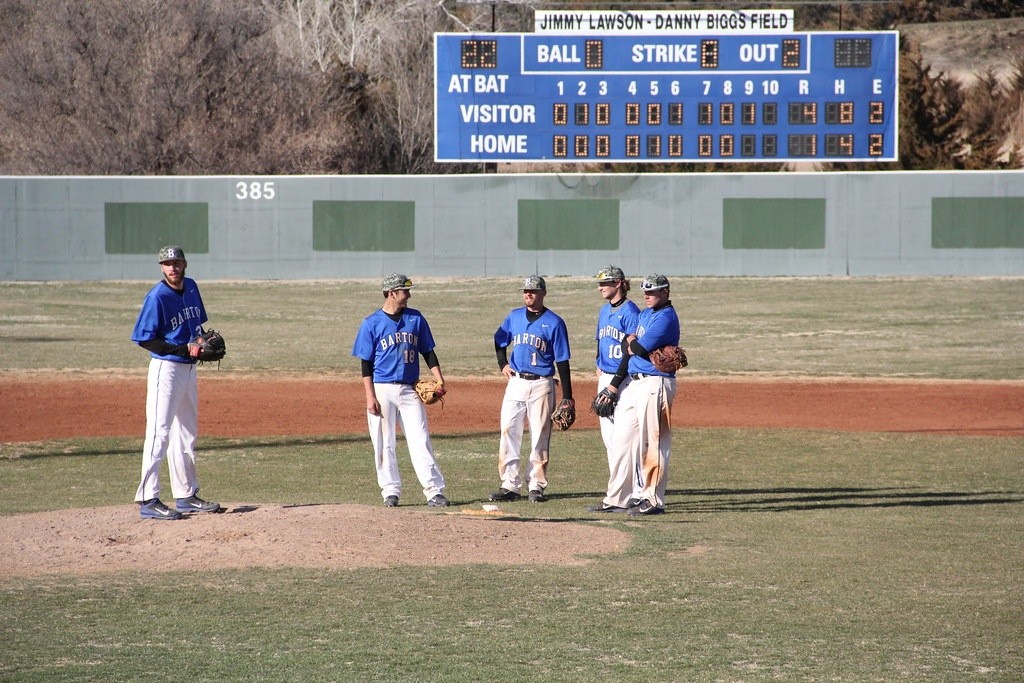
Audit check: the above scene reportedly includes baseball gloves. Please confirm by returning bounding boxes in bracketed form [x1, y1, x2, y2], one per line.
[188, 331, 225, 361]
[649, 346, 688, 372]
[592, 387, 618, 417]
[550, 399, 576, 431]
[413, 380, 446, 404]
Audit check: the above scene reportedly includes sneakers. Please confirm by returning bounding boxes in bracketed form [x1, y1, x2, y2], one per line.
[428, 494, 450, 507]
[529, 490, 544, 502]
[139, 498, 182, 520]
[625, 498, 664, 516]
[585, 502, 626, 513]
[384, 495, 398, 507]
[488, 488, 521, 502]
[176, 492, 220, 513]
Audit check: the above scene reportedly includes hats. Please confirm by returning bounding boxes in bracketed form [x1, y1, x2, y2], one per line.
[518, 275, 546, 291]
[597, 265, 625, 283]
[158, 245, 186, 264]
[640, 272, 669, 291]
[382, 272, 417, 292]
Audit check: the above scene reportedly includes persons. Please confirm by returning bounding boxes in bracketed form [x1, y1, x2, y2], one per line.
[131, 246, 220, 520]
[489, 275, 575, 503]
[624, 273, 688, 515]
[587, 265, 641, 513]
[352, 272, 450, 508]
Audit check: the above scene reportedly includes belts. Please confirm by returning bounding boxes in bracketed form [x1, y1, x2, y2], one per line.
[631, 373, 648, 380]
[510, 371, 547, 380]
